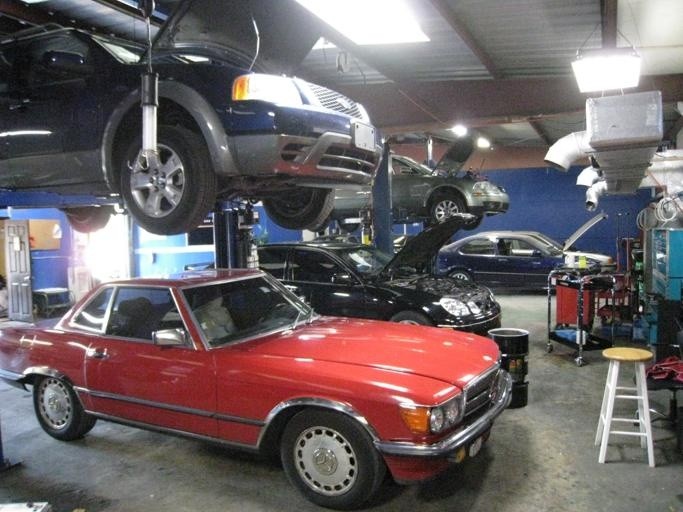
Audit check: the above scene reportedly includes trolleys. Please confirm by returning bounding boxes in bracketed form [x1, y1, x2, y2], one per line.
[545, 270, 616, 368]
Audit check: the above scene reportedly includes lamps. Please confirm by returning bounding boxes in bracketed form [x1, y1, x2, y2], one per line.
[572, 20, 645, 94]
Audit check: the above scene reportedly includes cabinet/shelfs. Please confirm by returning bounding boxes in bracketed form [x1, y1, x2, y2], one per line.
[650, 224, 683, 302]
[33, 286, 76, 320]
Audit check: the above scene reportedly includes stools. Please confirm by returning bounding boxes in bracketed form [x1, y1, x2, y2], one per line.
[595, 346, 659, 467]
[631, 371, 683, 433]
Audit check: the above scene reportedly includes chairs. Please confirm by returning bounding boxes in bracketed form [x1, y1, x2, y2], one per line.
[192, 285, 238, 342]
[118, 295, 162, 340]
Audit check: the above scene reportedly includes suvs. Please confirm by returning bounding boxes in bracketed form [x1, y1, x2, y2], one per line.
[1, 1, 382, 235]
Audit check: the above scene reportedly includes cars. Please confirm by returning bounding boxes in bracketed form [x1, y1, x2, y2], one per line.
[0, 269, 514, 509]
[309, 129, 510, 233]
[435, 212, 615, 293]
[185, 241, 502, 336]
